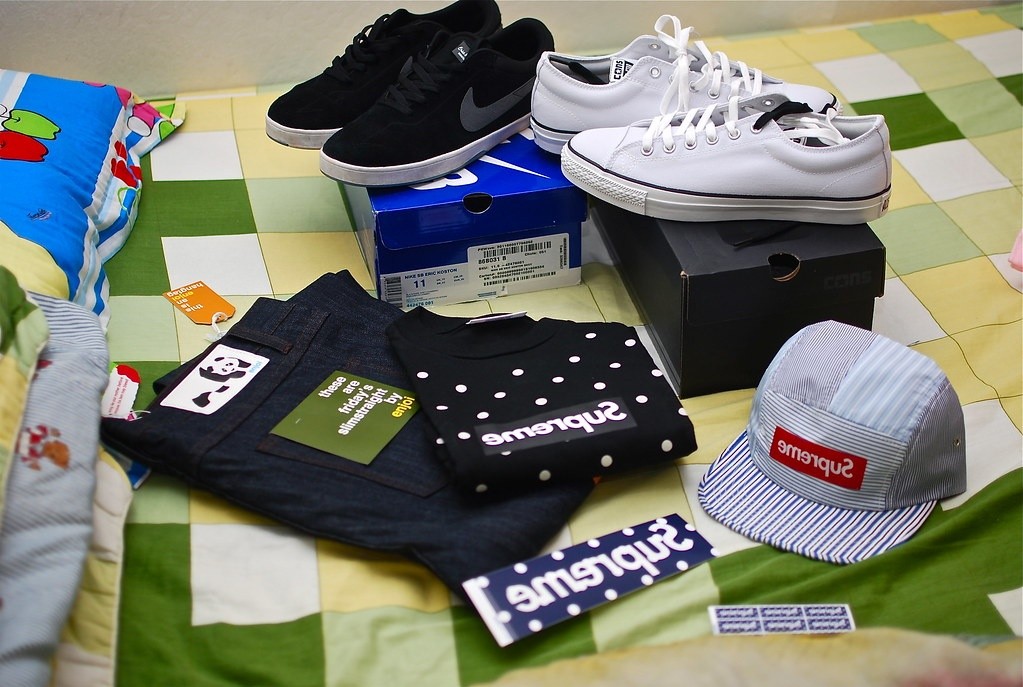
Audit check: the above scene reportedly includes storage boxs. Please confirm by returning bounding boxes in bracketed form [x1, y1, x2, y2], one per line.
[342, 126, 888, 386]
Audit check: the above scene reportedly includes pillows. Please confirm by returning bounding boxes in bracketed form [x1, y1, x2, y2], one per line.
[1, 67, 177, 311]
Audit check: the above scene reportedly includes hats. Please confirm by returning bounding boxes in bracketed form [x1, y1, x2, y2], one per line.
[696, 318, 966, 563]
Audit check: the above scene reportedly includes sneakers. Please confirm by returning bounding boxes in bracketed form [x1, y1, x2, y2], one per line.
[318, 15, 557, 187]
[266, 0, 505, 150]
[530, 31, 841, 160]
[560, 91, 892, 225]
